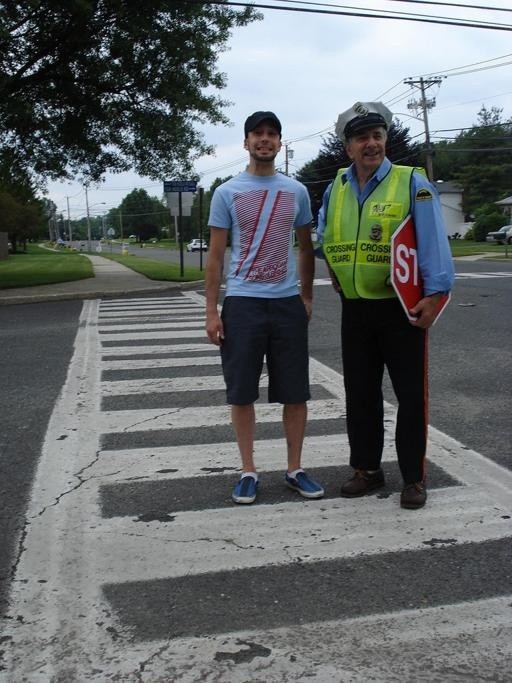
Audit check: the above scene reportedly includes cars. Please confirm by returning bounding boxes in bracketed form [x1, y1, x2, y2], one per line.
[185, 238, 208, 252]
[129, 234, 136, 238]
[78, 240, 86, 245]
[486, 224, 512, 244]
[55, 238, 65, 245]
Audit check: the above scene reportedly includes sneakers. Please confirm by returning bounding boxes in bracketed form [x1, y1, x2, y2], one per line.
[232, 474, 259, 504]
[285, 471, 324, 498]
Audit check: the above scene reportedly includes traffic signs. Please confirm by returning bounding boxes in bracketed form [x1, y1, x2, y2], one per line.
[163, 179, 196, 191]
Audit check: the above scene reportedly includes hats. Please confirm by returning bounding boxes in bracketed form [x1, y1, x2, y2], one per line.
[335, 101, 394, 140]
[245, 111, 281, 131]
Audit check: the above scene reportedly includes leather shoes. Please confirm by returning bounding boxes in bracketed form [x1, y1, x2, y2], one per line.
[341, 470, 384, 498]
[400, 481, 427, 509]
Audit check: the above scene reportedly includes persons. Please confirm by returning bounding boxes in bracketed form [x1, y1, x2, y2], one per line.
[205, 111, 325, 503]
[317, 102, 454, 509]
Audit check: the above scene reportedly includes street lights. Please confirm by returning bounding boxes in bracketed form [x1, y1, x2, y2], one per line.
[85, 201, 107, 251]
[48, 208, 66, 243]
[390, 111, 436, 190]
